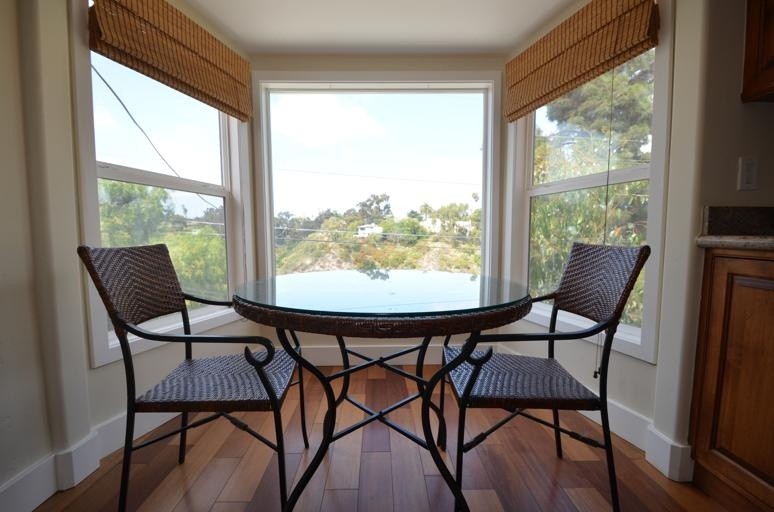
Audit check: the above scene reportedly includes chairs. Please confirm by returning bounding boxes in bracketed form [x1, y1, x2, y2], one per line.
[75, 244, 309, 511]
[437, 242, 651, 512]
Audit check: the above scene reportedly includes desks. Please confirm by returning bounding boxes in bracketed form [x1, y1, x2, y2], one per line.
[232, 269, 532, 512]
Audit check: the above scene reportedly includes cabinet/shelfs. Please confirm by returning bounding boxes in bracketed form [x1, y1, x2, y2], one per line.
[687, 249, 773, 512]
